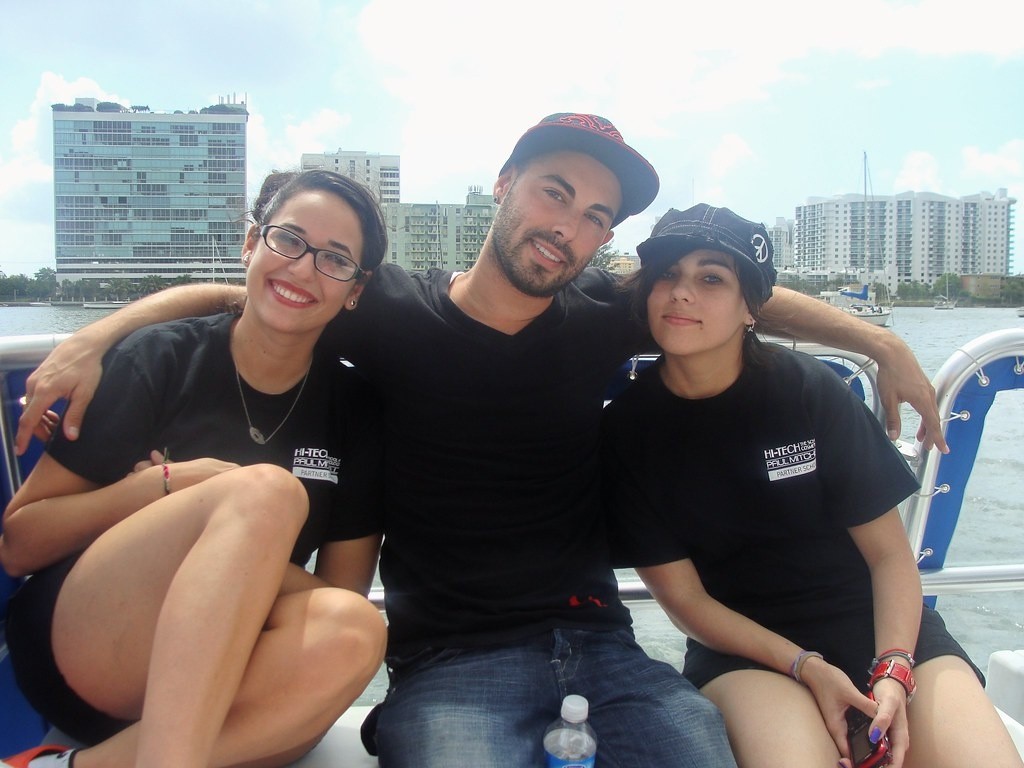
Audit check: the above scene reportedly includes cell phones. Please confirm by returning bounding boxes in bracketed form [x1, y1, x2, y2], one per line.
[845, 690, 893, 768]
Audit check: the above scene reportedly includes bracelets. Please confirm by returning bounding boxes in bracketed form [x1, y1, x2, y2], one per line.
[867, 660, 917, 707]
[163, 447, 169, 495]
[789, 649, 823, 687]
[871, 648, 914, 672]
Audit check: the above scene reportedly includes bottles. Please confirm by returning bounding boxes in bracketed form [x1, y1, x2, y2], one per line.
[542, 694, 598, 768]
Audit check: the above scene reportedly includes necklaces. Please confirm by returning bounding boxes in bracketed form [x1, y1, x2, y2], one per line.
[234, 355, 311, 445]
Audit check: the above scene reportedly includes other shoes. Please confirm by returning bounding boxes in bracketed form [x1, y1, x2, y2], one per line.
[0, 744, 73, 768]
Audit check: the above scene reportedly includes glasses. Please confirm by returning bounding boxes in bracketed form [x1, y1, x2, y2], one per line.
[259, 224, 365, 282]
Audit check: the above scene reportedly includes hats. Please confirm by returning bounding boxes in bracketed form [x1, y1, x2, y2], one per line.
[636, 203, 778, 314]
[498, 113, 659, 230]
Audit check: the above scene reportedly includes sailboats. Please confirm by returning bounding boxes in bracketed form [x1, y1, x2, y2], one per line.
[835, 151, 893, 326]
[934, 273, 955, 309]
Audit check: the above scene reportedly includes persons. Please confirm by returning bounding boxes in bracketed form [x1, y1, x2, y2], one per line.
[14, 112, 950, 768]
[602, 204, 1022, 768]
[1, 168, 389, 768]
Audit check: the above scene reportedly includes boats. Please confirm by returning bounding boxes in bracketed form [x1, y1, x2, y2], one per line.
[30, 302, 51, 307]
[83, 301, 129, 309]
[1015, 307, 1024, 318]
